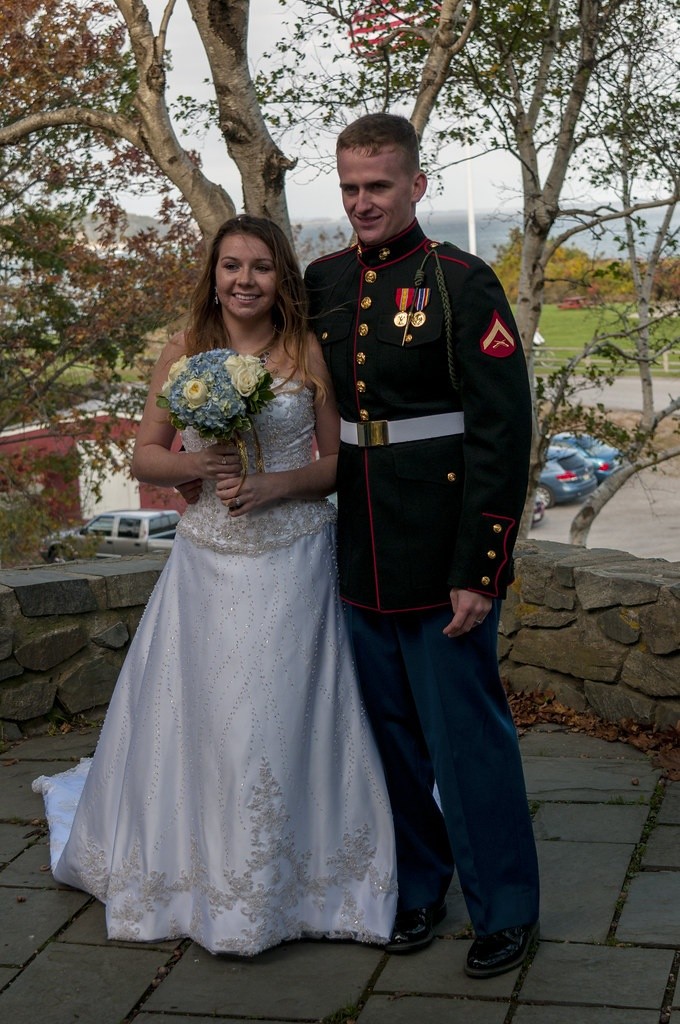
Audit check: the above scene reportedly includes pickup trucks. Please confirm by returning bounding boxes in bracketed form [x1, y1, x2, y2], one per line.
[38, 509, 183, 565]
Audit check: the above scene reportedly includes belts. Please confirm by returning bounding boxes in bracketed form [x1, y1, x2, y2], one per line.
[339, 411, 464, 446]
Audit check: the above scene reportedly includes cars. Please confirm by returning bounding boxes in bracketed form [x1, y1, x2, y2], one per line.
[548, 431, 624, 484]
[536, 443, 600, 511]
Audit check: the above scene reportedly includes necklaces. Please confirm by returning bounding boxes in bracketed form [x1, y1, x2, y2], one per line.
[259, 325, 276, 366]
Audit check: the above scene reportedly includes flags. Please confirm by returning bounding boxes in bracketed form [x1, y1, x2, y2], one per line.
[348, 0, 445, 57]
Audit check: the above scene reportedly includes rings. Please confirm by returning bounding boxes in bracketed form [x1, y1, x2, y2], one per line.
[235, 497, 241, 506]
[475, 620, 480, 624]
[221, 456, 226, 464]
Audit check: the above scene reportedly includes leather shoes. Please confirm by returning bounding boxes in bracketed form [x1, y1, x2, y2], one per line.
[465, 918, 540, 977]
[382, 898, 448, 951]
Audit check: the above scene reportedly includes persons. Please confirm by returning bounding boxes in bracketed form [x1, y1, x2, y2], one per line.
[31, 214, 401, 955]
[175, 113, 543, 979]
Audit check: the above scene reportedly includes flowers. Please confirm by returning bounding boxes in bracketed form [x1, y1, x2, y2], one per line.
[155, 348, 276, 442]
